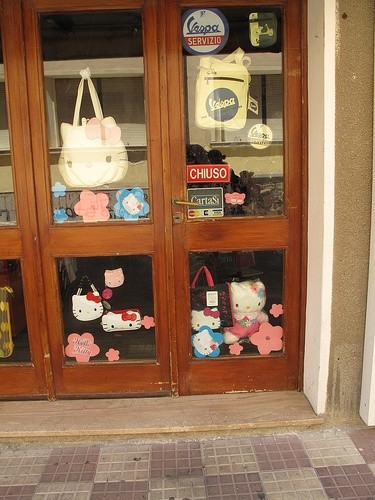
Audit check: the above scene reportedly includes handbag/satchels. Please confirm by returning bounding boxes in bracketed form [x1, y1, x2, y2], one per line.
[190, 266, 235, 332]
[58, 75, 130, 189]
[71, 276, 105, 323]
[194, 47, 251, 132]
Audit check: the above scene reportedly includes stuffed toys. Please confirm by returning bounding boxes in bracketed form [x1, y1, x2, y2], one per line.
[220, 278, 270, 346]
[187, 144, 260, 216]
